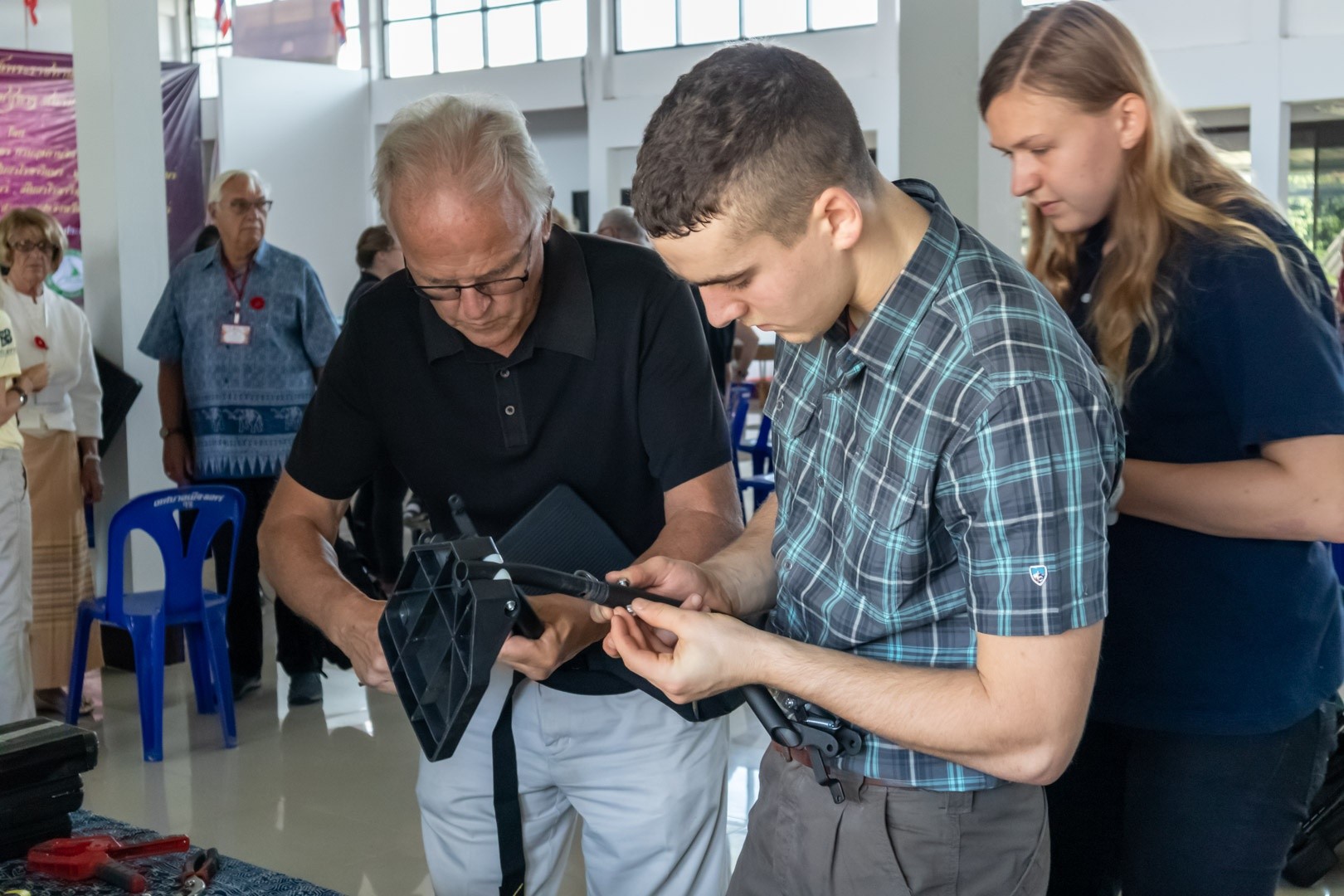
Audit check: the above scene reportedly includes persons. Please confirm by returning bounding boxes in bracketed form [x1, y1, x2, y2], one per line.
[0, 168, 738, 736]
[590, 37, 1131, 896]
[977, 0, 1344, 896]
[254, 95, 742, 896]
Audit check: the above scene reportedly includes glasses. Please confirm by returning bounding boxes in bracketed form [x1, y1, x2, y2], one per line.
[9, 238, 53, 253]
[403, 227, 532, 301]
[229, 198, 273, 215]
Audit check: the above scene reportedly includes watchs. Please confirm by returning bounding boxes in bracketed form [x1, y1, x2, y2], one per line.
[8, 385, 29, 408]
[159, 425, 183, 438]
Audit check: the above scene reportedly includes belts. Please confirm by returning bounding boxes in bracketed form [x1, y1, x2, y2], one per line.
[770, 738, 921, 788]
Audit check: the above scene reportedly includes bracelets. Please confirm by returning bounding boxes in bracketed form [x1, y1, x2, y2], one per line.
[83, 453, 101, 465]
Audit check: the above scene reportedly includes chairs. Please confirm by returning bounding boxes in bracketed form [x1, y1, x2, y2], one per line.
[63, 486, 247, 763]
[726, 382, 775, 516]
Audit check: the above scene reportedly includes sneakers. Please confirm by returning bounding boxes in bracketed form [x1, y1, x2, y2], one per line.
[288, 666, 329, 704]
[229, 671, 262, 700]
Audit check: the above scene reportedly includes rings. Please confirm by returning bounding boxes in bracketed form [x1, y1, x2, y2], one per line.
[166, 471, 172, 477]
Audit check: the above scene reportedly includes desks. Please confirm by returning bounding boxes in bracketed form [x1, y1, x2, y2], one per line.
[0, 809, 349, 896]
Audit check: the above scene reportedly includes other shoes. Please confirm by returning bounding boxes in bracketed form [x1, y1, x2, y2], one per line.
[35, 693, 95, 716]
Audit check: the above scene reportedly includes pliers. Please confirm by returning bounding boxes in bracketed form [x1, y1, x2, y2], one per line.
[173, 848, 219, 896]
[26, 834, 190, 893]
